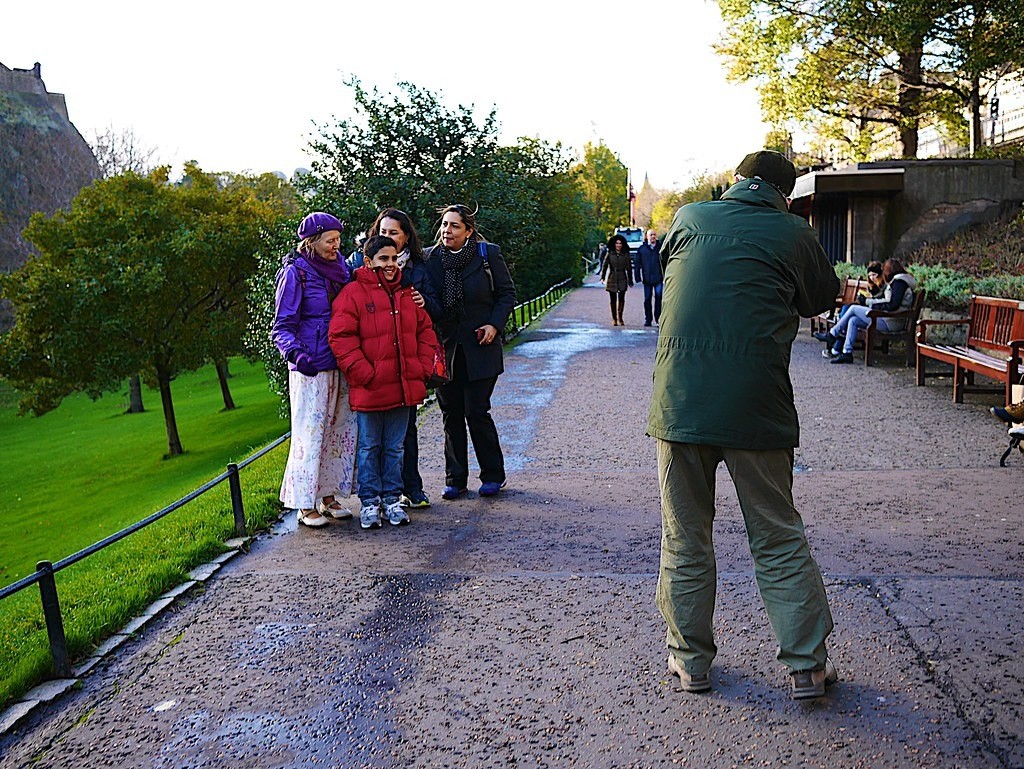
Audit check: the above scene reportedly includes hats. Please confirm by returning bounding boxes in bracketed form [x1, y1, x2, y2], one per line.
[736, 150, 796, 196]
[297, 212, 344, 238]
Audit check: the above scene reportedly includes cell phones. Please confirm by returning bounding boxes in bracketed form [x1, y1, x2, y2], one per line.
[478, 330, 486, 341]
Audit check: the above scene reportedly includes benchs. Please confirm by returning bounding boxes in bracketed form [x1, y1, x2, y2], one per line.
[810, 275, 926, 367]
[916, 295, 1024, 407]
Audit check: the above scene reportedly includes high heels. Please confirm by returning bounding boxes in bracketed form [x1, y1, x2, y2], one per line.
[319, 499, 352, 520]
[297, 508, 330, 529]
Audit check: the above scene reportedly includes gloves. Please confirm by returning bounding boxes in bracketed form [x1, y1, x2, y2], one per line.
[856, 293, 867, 306]
[292, 351, 318, 377]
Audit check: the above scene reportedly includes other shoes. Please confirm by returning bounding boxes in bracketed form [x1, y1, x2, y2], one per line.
[643, 320, 651, 326]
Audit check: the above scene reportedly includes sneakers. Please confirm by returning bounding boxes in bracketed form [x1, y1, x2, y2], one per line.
[830, 353, 853, 364]
[478, 477, 507, 496]
[813, 331, 836, 346]
[822, 348, 840, 358]
[360, 503, 383, 529]
[382, 502, 410, 527]
[667, 653, 712, 693]
[441, 485, 469, 500]
[791, 657, 838, 698]
[401, 490, 431, 509]
[989, 398, 1024, 424]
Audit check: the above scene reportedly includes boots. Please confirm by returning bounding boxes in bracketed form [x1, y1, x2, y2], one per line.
[610, 303, 617, 326]
[618, 302, 624, 325]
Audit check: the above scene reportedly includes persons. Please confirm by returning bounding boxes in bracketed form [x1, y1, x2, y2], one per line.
[645, 151, 837, 700]
[635, 229, 664, 327]
[813, 258, 916, 363]
[327, 236, 438, 529]
[600, 235, 634, 326]
[345, 208, 440, 507]
[422, 204, 517, 499]
[271, 212, 359, 528]
[596, 243, 607, 275]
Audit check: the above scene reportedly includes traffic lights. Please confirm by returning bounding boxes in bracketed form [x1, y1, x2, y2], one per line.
[990, 98, 999, 119]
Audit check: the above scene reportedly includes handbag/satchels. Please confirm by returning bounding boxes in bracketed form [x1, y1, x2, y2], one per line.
[419, 337, 466, 392]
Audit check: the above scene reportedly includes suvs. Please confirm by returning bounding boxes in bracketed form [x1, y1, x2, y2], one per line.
[614, 226, 644, 264]
